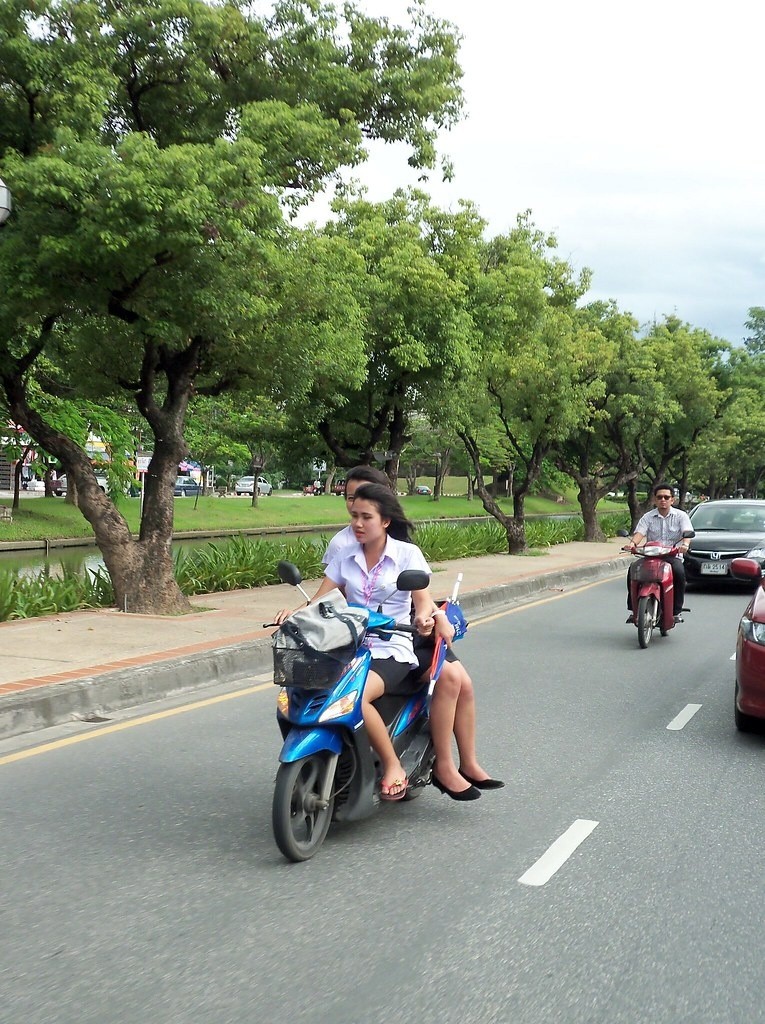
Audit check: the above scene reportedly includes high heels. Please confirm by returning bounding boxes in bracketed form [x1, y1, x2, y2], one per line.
[430, 760, 481, 801]
[458, 768, 505, 790]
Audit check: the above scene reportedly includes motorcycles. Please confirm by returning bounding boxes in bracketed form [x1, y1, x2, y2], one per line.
[615, 528, 694, 649]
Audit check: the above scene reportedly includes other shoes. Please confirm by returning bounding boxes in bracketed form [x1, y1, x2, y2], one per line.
[626, 615, 633, 623]
[674, 615, 679, 623]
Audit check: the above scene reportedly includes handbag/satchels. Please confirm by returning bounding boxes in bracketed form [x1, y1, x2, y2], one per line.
[270, 588, 369, 651]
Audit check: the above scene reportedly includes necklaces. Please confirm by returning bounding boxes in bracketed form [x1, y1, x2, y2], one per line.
[361, 555, 388, 606]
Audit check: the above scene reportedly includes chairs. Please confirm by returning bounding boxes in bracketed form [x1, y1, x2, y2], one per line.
[718, 514, 733, 527]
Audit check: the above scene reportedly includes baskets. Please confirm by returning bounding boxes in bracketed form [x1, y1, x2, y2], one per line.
[630, 560, 664, 582]
[272, 645, 355, 689]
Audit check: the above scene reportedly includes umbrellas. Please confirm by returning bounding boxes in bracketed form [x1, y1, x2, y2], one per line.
[427, 572, 469, 695]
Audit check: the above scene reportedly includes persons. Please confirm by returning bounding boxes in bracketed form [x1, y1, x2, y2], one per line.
[49, 469, 57, 480]
[21, 473, 41, 489]
[623, 484, 694, 624]
[274, 483, 435, 800]
[321, 466, 504, 802]
[314, 478, 323, 496]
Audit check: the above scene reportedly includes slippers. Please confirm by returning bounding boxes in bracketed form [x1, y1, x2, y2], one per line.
[380, 776, 409, 799]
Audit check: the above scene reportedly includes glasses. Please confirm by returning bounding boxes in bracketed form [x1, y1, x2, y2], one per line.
[656, 495, 671, 500]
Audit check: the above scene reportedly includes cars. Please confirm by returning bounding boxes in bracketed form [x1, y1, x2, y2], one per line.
[678, 500, 765, 588]
[54, 468, 125, 496]
[172, 476, 203, 497]
[235, 476, 273, 496]
[416, 486, 431, 495]
[729, 557, 764, 732]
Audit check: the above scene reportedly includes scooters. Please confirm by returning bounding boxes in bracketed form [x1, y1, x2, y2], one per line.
[262, 559, 439, 863]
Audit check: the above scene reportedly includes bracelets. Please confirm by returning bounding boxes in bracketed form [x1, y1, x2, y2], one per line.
[431, 610, 445, 617]
